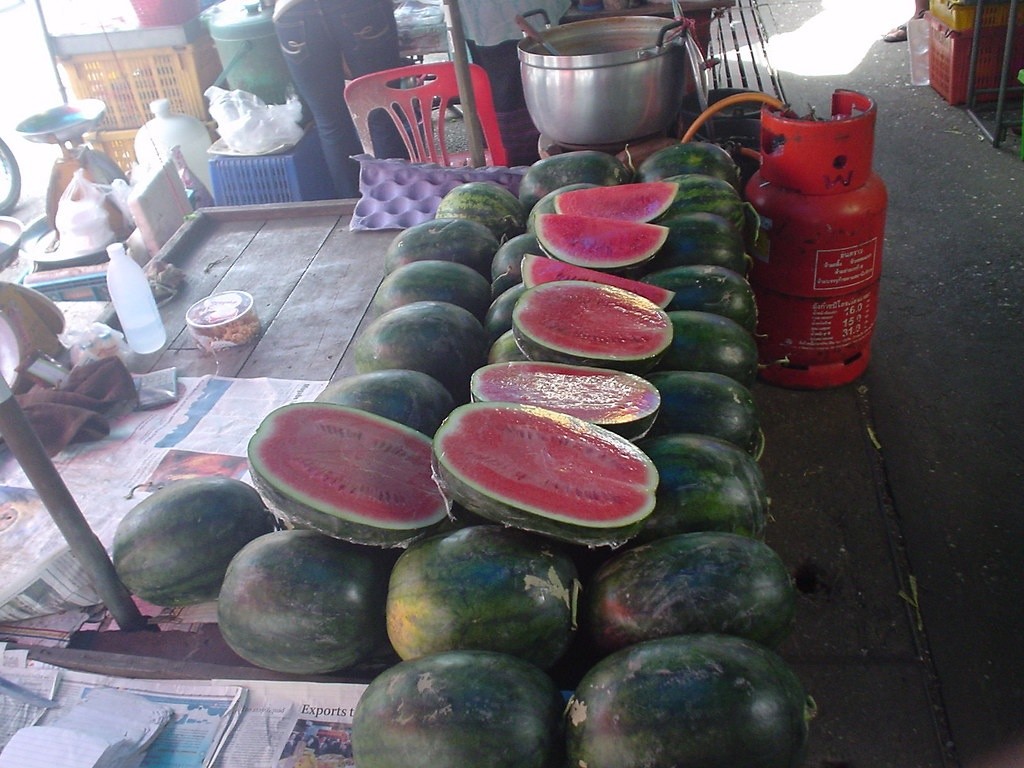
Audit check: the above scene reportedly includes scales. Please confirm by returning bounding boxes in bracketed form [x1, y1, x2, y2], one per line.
[13, 99, 131, 244]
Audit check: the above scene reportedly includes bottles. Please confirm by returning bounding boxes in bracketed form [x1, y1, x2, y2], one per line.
[107, 242, 167, 355]
[133, 98, 213, 201]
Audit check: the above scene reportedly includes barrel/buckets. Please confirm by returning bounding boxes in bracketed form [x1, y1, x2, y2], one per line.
[683, 88, 776, 195]
[205, 0, 312, 126]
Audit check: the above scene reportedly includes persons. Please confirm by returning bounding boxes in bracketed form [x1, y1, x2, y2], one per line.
[272, 0, 417, 200]
[444, 0, 572, 167]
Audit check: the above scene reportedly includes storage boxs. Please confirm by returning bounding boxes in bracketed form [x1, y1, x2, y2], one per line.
[208, 126, 334, 207]
[16, 271, 112, 302]
[924, 0, 1024, 106]
[49, 13, 230, 177]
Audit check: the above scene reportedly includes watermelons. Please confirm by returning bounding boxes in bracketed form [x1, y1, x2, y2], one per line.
[217, 141, 816, 768]
[110, 475, 278, 608]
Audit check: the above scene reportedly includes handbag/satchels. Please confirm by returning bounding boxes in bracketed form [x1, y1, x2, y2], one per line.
[205, 85, 303, 149]
[55, 169, 135, 256]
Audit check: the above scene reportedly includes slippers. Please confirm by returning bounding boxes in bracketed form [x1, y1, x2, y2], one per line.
[882, 25, 908, 42]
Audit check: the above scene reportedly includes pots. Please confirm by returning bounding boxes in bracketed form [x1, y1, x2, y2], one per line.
[400, 57, 421, 122]
[516, 8, 689, 144]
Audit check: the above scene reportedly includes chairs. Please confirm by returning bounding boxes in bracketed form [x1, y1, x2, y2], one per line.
[343, 61, 510, 168]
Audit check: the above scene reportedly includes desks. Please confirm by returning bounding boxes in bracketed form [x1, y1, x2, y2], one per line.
[560, 0, 736, 97]
